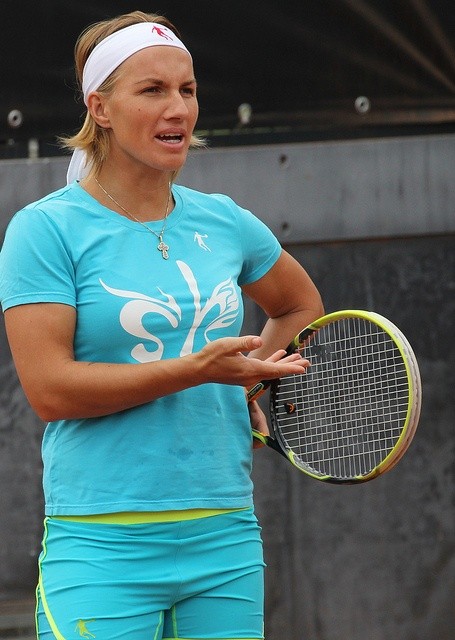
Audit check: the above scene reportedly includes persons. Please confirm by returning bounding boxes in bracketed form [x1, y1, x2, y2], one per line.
[0, 12, 325, 640]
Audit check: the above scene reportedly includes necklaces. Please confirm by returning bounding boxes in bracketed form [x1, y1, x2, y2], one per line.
[89, 176, 172, 260]
